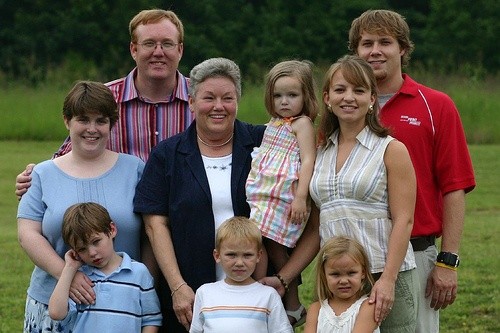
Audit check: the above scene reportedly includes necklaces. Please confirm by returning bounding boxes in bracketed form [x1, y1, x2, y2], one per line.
[197, 134, 234, 147]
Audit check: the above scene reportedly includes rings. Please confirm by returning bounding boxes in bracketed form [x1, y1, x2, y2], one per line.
[389, 307, 392, 311]
[75, 291, 80, 296]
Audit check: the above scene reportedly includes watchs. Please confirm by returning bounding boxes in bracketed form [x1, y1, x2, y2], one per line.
[436, 251, 460, 268]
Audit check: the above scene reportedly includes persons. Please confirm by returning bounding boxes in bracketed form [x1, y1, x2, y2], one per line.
[189, 217, 295, 333]
[16, 11, 196, 197]
[47, 200, 164, 333]
[244, 59, 318, 328]
[303, 236, 381, 332]
[133, 57, 303, 333]
[15, 80, 165, 333]
[347, 10, 477, 333]
[309, 55, 418, 333]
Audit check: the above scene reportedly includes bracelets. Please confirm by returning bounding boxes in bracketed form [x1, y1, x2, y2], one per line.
[435, 262, 457, 272]
[273, 273, 291, 293]
[171, 283, 186, 296]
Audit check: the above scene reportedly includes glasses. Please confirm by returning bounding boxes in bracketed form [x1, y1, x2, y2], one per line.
[137, 41, 181, 51]
